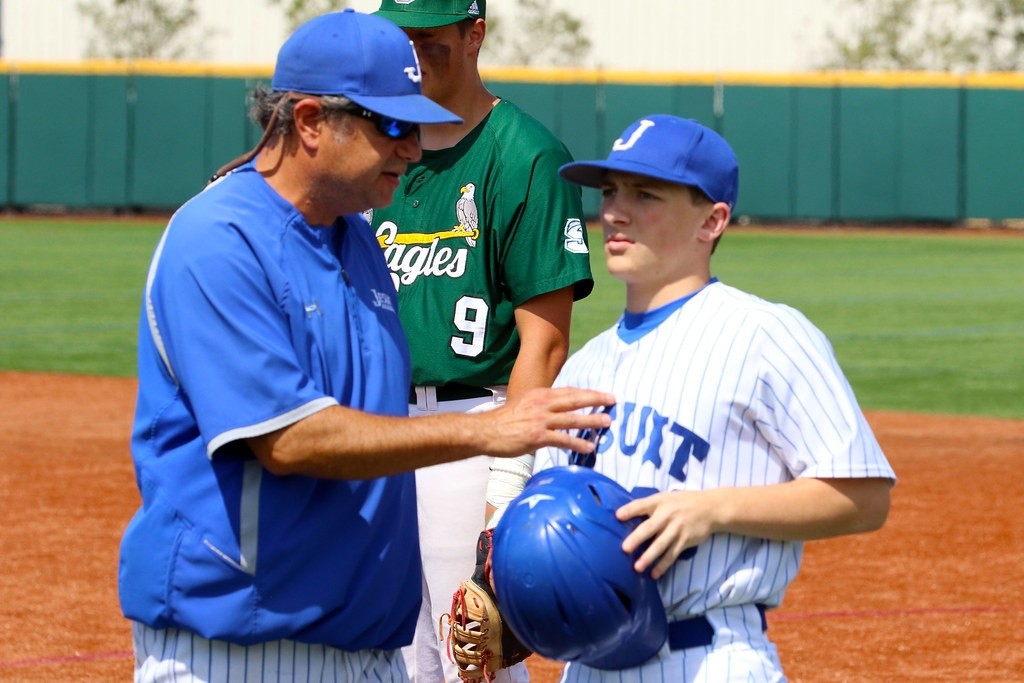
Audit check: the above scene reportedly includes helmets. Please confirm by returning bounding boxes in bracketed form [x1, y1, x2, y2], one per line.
[490, 465, 668, 672]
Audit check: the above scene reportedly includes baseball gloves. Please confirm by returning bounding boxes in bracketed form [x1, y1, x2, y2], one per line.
[437, 524, 531, 682]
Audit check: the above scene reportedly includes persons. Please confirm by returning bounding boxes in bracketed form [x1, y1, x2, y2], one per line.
[370, 0, 594, 683]
[439, 114, 897, 683]
[119, 8, 615, 683]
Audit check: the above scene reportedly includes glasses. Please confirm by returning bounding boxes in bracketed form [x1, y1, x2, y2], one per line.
[288, 97, 421, 139]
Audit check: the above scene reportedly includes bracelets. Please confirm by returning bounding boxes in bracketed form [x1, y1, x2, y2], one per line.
[489, 466, 532, 479]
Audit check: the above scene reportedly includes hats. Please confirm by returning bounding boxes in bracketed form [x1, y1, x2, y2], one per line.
[369, 0, 486, 28]
[559, 114, 740, 214]
[272, 7, 464, 126]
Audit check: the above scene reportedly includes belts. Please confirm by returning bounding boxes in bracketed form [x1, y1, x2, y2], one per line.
[666, 603, 767, 653]
[407, 384, 493, 405]
